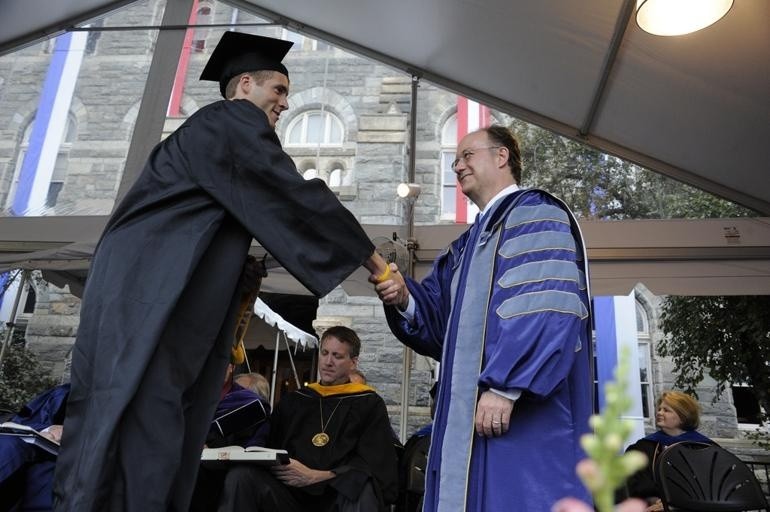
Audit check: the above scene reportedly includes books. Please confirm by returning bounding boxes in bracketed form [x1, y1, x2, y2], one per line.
[200, 446, 289, 467]
[0, 421, 60, 456]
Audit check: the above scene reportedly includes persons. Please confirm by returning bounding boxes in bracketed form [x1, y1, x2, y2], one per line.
[392, 381, 438, 512]
[188, 356, 271, 512]
[216, 326, 399, 512]
[369, 125, 594, 512]
[233, 373, 270, 405]
[349, 371, 368, 386]
[623, 391, 735, 512]
[49, 31, 405, 512]
[0, 381, 70, 512]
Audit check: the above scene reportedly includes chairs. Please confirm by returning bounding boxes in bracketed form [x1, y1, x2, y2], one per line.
[398, 429, 431, 512]
[656, 438, 770, 510]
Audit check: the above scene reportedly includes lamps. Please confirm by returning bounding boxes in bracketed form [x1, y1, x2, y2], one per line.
[632, 0, 737, 36]
[395, 181, 422, 201]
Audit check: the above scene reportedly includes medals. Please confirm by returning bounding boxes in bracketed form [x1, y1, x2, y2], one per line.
[311, 432, 330, 448]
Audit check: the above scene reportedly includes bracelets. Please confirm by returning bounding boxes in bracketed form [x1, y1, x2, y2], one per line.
[376, 263, 390, 283]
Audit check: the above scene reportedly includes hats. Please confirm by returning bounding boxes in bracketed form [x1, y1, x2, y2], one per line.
[198, 31, 294, 100]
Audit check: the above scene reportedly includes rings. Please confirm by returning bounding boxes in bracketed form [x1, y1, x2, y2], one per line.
[493, 421, 501, 424]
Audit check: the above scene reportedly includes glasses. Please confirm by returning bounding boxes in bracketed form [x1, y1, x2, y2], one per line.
[451, 146, 501, 171]
